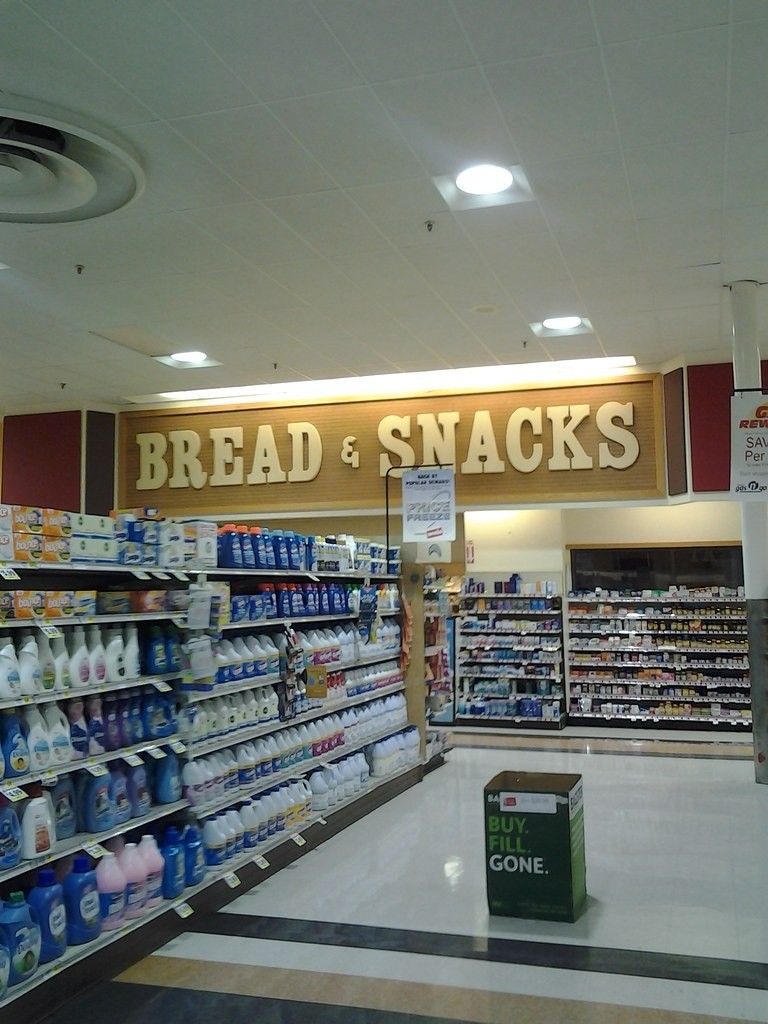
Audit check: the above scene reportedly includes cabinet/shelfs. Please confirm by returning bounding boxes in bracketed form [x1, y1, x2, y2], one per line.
[457, 593, 570, 731]
[421, 589, 458, 724]
[0, 504, 457, 1024]
[563, 596, 753, 733]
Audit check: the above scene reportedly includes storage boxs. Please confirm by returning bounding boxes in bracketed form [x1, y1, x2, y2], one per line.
[484, 771, 587, 923]
[0, 502, 230, 624]
[459, 574, 563, 716]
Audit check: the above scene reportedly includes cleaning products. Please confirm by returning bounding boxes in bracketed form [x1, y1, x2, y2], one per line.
[0, 504, 454, 1006]
